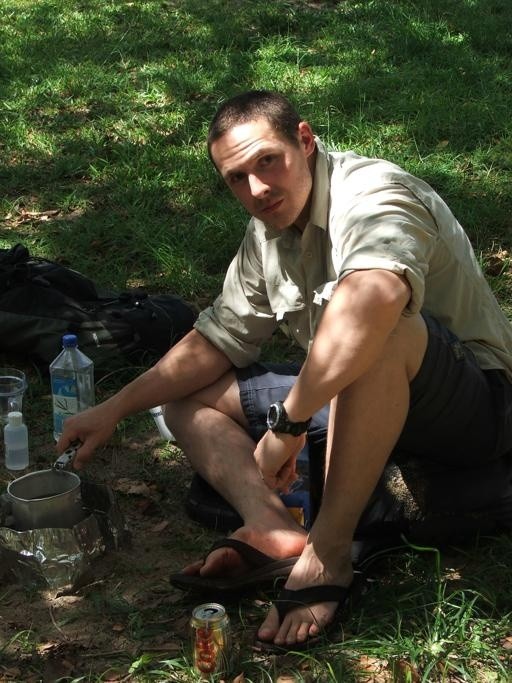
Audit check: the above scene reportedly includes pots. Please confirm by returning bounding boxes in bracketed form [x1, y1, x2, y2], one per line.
[8, 443, 84, 529]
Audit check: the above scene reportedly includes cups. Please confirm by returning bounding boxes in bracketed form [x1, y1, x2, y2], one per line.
[0, 368, 28, 426]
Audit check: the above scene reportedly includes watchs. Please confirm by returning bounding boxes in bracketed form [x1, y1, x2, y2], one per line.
[266, 397, 312, 436]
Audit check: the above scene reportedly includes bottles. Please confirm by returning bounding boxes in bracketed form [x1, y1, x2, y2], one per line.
[4, 336, 95, 471]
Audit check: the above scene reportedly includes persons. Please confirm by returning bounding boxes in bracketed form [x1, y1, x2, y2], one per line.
[53, 87, 510, 645]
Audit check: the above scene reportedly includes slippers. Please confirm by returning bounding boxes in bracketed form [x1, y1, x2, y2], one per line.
[170, 537, 302, 592]
[257, 570, 362, 650]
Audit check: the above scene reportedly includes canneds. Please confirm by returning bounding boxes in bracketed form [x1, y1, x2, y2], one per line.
[189, 603, 232, 679]
